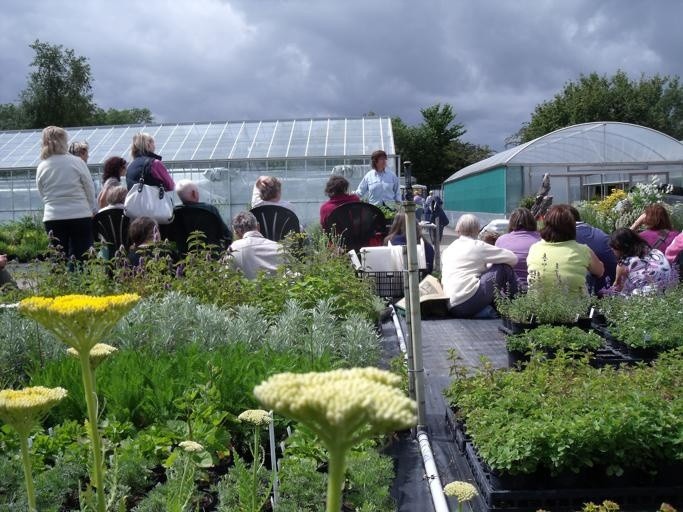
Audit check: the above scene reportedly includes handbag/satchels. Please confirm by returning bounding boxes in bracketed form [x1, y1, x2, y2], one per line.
[123, 182, 176, 225]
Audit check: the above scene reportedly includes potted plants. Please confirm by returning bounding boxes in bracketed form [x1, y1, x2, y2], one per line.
[445, 342, 682, 511]
[488, 251, 680, 366]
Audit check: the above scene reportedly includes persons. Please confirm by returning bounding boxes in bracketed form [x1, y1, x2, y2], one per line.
[36, 126, 98, 265]
[251, 175, 304, 233]
[352, 151, 402, 209]
[320, 176, 358, 224]
[496, 207, 543, 293]
[0, 250, 18, 294]
[96, 133, 233, 272]
[413, 191, 435, 212]
[611, 203, 683, 295]
[526, 205, 616, 317]
[224, 210, 294, 280]
[441, 214, 517, 317]
[385, 212, 434, 276]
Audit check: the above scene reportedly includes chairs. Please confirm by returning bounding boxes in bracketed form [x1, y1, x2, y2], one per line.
[164, 206, 230, 260]
[322, 201, 385, 250]
[247, 204, 300, 243]
[356, 244, 422, 300]
[92, 207, 132, 259]
